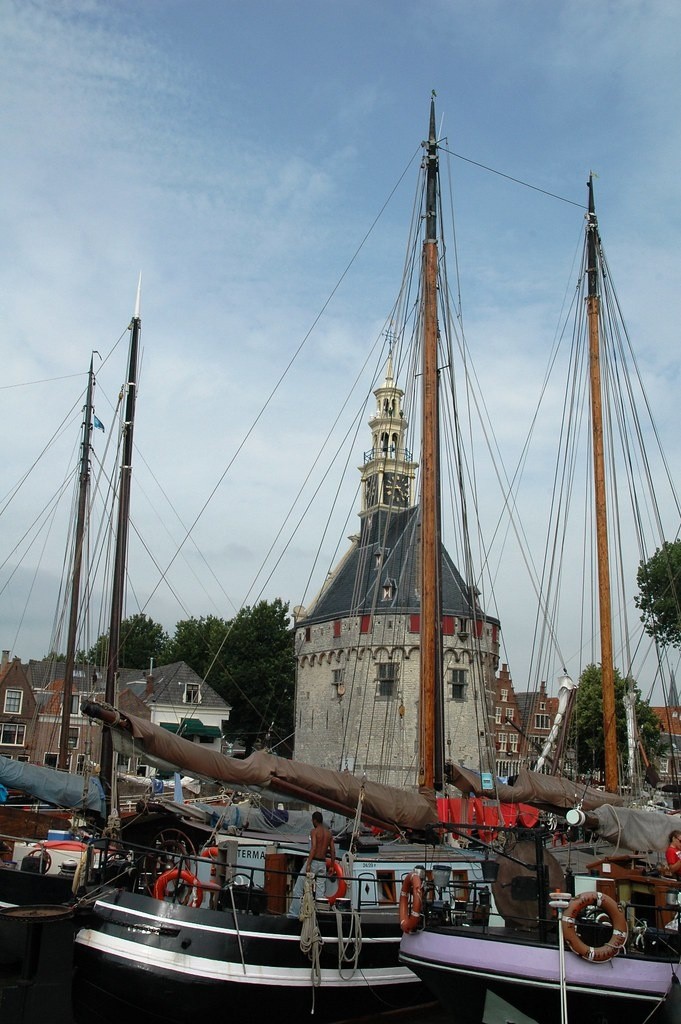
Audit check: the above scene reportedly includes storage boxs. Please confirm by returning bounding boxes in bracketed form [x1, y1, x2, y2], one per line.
[48, 829, 73, 840]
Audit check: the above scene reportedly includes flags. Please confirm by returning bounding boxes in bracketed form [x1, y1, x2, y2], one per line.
[93, 415, 104, 433]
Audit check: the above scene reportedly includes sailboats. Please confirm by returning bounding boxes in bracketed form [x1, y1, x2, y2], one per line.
[0, 91, 679, 1023]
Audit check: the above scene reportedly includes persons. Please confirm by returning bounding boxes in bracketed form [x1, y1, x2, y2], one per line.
[665, 830, 681, 881]
[286, 811, 336, 919]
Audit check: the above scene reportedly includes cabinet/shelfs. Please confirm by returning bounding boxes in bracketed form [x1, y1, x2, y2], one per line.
[217, 839, 239, 887]
[265, 853, 308, 914]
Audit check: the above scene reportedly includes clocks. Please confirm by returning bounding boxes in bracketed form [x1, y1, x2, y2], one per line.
[366, 473, 378, 508]
[383, 471, 408, 507]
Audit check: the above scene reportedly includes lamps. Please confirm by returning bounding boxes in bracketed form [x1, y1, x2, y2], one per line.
[432, 865, 452, 887]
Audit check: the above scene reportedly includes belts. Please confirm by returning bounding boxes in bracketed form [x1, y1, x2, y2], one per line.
[313, 857, 326, 862]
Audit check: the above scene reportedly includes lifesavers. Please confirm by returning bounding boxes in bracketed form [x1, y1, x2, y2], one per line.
[70, 850, 95, 893]
[560, 889, 632, 967]
[151, 867, 205, 910]
[397, 869, 427, 936]
[199, 845, 222, 894]
[321, 857, 350, 909]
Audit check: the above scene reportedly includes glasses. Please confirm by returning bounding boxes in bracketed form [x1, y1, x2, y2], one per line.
[674, 836, 681, 842]
